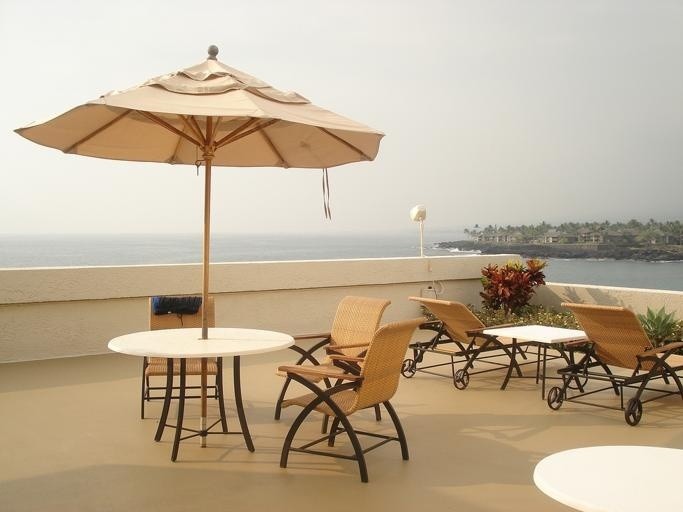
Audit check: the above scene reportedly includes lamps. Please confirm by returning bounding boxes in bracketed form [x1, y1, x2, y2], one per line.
[410, 205, 426, 259]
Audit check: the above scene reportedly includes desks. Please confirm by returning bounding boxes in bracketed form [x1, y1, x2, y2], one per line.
[108, 327, 295, 462]
[533, 445, 683, 511]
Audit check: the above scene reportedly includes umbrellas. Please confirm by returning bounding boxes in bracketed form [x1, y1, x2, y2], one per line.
[12, 47, 387, 448]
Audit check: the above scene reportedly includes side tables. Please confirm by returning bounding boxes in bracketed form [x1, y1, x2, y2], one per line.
[483, 325, 589, 399]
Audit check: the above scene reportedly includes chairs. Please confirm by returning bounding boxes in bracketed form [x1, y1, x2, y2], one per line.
[401, 296, 564, 390]
[548, 302, 683, 426]
[280, 316, 427, 483]
[141, 296, 219, 419]
[275, 295, 392, 433]
